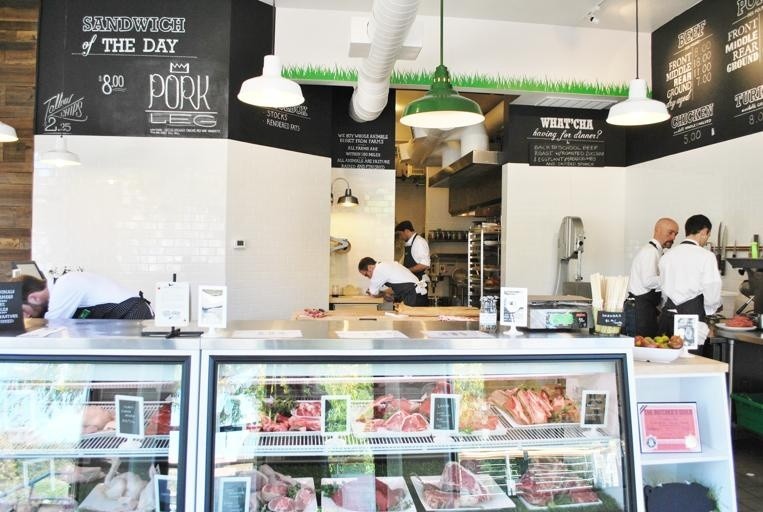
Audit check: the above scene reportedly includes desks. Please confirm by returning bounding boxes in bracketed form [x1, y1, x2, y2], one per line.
[712, 325, 763, 438]
[328, 295, 384, 312]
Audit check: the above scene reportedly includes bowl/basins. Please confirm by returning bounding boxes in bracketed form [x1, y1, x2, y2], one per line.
[452, 268, 468, 283]
[482, 241, 499, 246]
[635, 345, 683, 364]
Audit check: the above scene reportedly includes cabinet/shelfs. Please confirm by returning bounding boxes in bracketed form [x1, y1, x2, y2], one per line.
[464, 229, 500, 306]
[194, 320, 647, 512]
[635, 351, 737, 512]
[0, 318, 199, 512]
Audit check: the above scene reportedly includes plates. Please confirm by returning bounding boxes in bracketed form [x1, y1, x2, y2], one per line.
[349, 400, 507, 441]
[243, 399, 323, 436]
[248, 474, 318, 512]
[491, 400, 581, 432]
[509, 476, 603, 510]
[409, 473, 516, 510]
[321, 476, 417, 511]
[716, 324, 757, 332]
[30, 402, 170, 512]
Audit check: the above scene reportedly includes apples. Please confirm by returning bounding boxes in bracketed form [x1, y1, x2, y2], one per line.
[635, 336, 686, 349]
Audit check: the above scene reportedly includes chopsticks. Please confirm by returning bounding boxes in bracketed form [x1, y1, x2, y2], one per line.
[590, 274, 629, 333]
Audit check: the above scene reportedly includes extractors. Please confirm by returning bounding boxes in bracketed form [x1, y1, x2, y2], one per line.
[429, 121, 501, 217]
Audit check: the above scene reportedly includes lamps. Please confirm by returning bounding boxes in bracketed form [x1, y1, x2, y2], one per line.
[329, 175, 358, 207]
[233, 1, 305, 111]
[397, 1, 487, 131]
[603, 1, 670, 129]
[0, 120, 18, 143]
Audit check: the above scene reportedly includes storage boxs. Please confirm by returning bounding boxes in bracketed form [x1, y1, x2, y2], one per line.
[730, 389, 763, 435]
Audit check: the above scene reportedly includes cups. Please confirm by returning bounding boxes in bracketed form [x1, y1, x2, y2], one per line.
[592, 306, 624, 337]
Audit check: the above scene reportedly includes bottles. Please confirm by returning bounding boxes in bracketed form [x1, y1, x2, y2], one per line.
[751, 233, 760, 260]
[332, 285, 338, 297]
[479, 296, 499, 332]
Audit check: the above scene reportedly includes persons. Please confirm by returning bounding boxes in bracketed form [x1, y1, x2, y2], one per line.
[395, 219, 432, 289]
[658, 214, 725, 354]
[15, 274, 155, 325]
[624, 217, 679, 336]
[358, 258, 428, 305]
